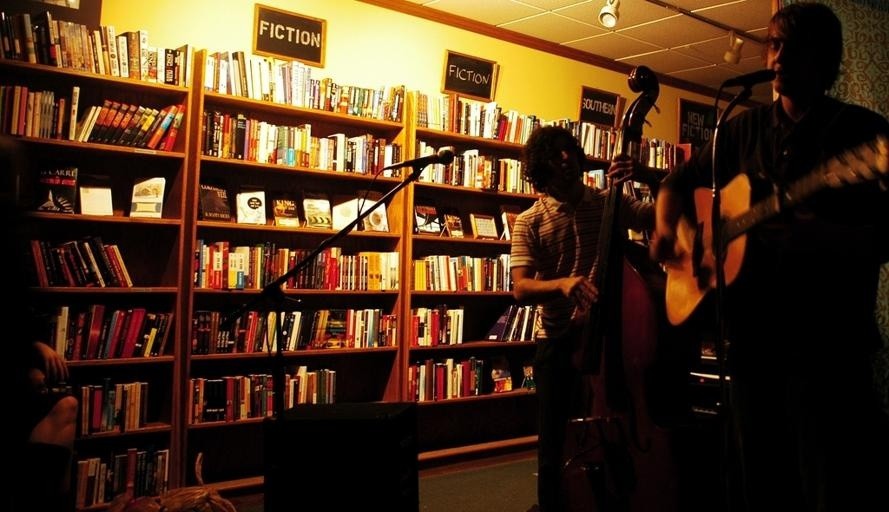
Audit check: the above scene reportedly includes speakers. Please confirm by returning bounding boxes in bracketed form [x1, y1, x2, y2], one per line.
[263, 401, 420, 512]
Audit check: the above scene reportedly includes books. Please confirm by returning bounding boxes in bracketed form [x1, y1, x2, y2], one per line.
[547, 119, 687, 245]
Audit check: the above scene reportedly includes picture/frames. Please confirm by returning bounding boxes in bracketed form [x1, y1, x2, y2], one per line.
[579, 86, 623, 130]
[677, 97, 720, 146]
[1, 0, 103, 28]
[441, 48, 500, 103]
[252, 1, 328, 69]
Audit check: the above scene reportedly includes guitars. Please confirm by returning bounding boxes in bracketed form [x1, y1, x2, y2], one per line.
[664, 143, 867, 325]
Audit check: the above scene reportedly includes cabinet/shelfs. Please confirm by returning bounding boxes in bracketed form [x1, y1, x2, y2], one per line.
[181, 50, 407, 490]
[408, 89, 691, 460]
[1, 45, 195, 512]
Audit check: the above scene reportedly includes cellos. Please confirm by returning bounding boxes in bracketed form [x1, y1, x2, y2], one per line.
[559, 65, 711, 512]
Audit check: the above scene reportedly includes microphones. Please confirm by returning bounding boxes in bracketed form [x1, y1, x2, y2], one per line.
[383, 146, 454, 170]
[724, 68, 776, 88]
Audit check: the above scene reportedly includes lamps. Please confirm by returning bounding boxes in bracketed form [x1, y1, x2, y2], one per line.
[722, 32, 744, 64]
[598, 0, 621, 28]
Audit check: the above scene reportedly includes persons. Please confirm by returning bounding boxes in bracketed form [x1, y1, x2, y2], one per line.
[649, 1, 887, 511]
[509, 124, 671, 511]
